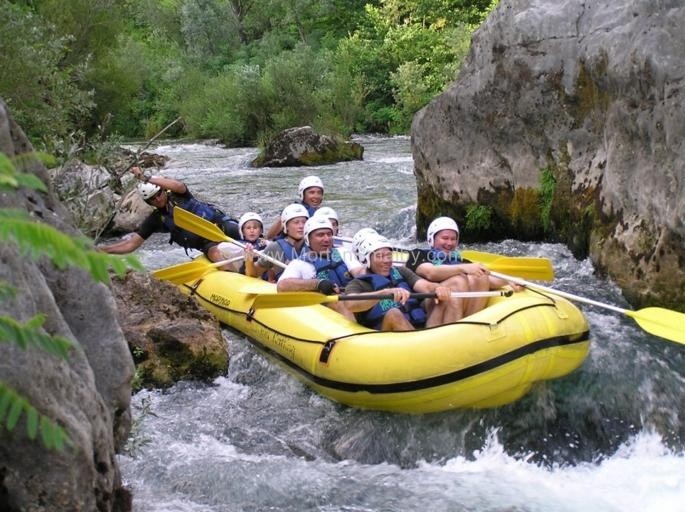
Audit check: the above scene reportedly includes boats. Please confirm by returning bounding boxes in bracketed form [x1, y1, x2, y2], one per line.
[177, 255, 592, 414]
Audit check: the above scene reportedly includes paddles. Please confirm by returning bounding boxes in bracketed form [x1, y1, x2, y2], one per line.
[172, 205, 288, 270]
[150, 250, 264, 285]
[393, 257, 555, 282]
[245, 288, 514, 309]
[487, 270, 684, 346]
[332, 235, 501, 265]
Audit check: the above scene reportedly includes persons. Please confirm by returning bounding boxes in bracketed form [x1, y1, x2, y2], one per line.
[405, 217, 527, 321]
[343, 235, 463, 332]
[245, 204, 309, 285]
[276, 214, 367, 325]
[312, 206, 339, 237]
[238, 210, 273, 253]
[267, 174, 325, 239]
[94, 166, 246, 271]
[348, 228, 380, 276]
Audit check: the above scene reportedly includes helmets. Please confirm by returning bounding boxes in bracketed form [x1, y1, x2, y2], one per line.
[314, 208, 339, 230]
[427, 217, 459, 250]
[138, 182, 162, 202]
[238, 211, 264, 240]
[298, 176, 324, 200]
[303, 217, 335, 247]
[281, 204, 310, 234]
[361, 234, 395, 269]
[352, 230, 382, 259]
